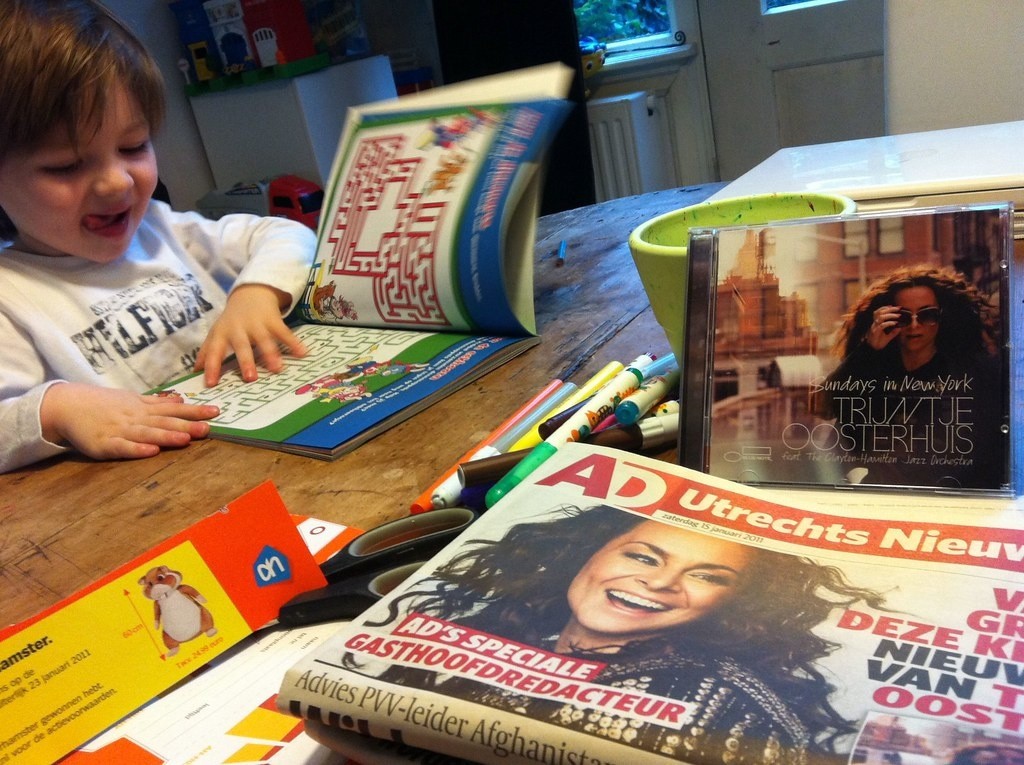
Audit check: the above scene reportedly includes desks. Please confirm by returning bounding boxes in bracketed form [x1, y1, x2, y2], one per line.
[0, 180, 1024, 765]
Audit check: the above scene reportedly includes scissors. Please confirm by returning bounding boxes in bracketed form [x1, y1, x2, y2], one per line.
[253, 506, 483, 629]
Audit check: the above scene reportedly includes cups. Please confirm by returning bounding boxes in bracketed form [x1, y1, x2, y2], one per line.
[631, 193, 847, 358]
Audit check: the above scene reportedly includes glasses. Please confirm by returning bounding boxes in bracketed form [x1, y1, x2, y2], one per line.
[892, 307, 943, 328]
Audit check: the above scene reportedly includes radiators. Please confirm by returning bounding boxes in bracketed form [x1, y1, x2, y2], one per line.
[586, 91, 670, 204]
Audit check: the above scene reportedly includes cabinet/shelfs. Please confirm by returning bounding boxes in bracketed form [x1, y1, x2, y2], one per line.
[183, 38, 398, 191]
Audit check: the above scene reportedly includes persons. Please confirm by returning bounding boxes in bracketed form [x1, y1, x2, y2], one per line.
[375, 498, 885, 765]
[811, 265, 1008, 488]
[945, 741, 1024, 765]
[0, 0, 320, 483]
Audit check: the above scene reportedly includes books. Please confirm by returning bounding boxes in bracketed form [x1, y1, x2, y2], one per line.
[140, 56, 576, 462]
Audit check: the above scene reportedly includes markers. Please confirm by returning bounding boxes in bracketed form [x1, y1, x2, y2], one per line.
[412, 351, 681, 511]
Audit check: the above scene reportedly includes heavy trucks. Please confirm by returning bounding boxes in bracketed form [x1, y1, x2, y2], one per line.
[195, 174, 324, 234]
